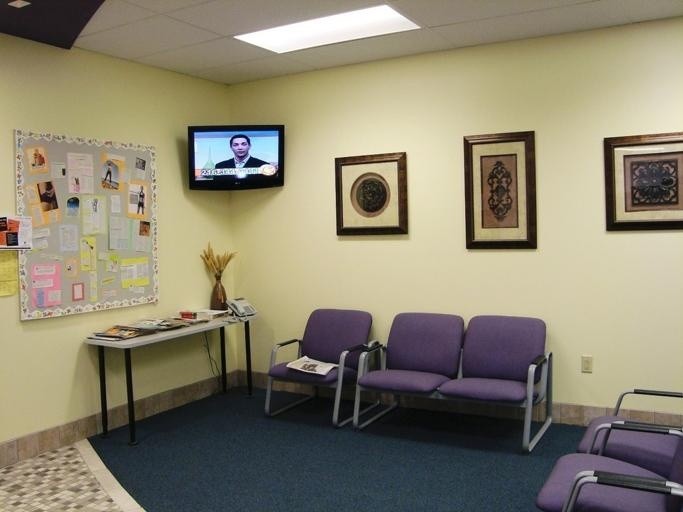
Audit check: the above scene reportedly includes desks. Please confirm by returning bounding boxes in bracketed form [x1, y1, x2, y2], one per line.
[84, 306, 256, 443]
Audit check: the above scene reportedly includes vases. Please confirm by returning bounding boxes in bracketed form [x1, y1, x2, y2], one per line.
[209, 274, 228, 310]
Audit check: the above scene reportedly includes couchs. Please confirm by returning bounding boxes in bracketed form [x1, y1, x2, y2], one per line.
[261, 306, 383, 429]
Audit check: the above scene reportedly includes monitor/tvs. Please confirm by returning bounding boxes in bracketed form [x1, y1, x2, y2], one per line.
[188, 125, 284, 191]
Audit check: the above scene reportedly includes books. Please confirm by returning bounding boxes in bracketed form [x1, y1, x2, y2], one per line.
[86, 309, 229, 343]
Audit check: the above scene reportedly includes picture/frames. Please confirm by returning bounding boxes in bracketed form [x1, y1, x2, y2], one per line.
[334, 152, 408, 238]
[603, 132, 683, 232]
[462, 130, 539, 251]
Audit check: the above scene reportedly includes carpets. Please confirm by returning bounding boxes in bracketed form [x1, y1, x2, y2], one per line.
[1, 438, 146, 511]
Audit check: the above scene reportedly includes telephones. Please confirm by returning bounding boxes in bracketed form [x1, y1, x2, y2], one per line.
[226, 297, 258, 317]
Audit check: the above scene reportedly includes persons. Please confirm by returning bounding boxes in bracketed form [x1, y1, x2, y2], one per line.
[103, 162, 115, 183]
[212, 134, 270, 180]
[129, 185, 145, 214]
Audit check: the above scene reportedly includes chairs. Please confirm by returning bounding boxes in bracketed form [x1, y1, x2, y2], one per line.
[534, 434, 683, 512]
[576, 388, 683, 475]
[353, 311, 555, 452]
